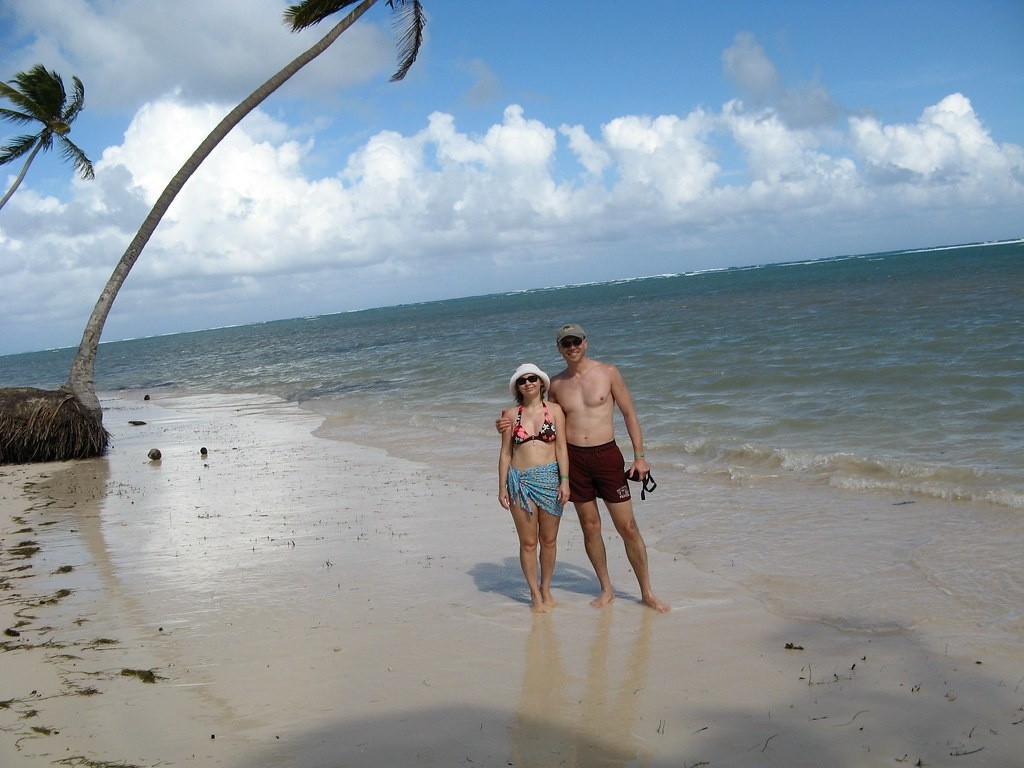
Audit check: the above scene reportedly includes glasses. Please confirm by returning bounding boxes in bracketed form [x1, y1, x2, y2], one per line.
[516, 375, 541, 386]
[558, 337, 586, 348]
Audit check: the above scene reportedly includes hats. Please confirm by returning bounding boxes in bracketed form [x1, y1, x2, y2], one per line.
[509, 363, 550, 397]
[556, 323, 587, 347]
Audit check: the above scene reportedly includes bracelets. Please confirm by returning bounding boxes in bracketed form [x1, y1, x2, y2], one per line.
[559, 475, 570, 479]
[633, 452, 644, 457]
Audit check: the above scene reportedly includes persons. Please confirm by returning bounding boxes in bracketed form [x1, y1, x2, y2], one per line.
[498, 364, 570, 613]
[495, 323, 671, 612]
